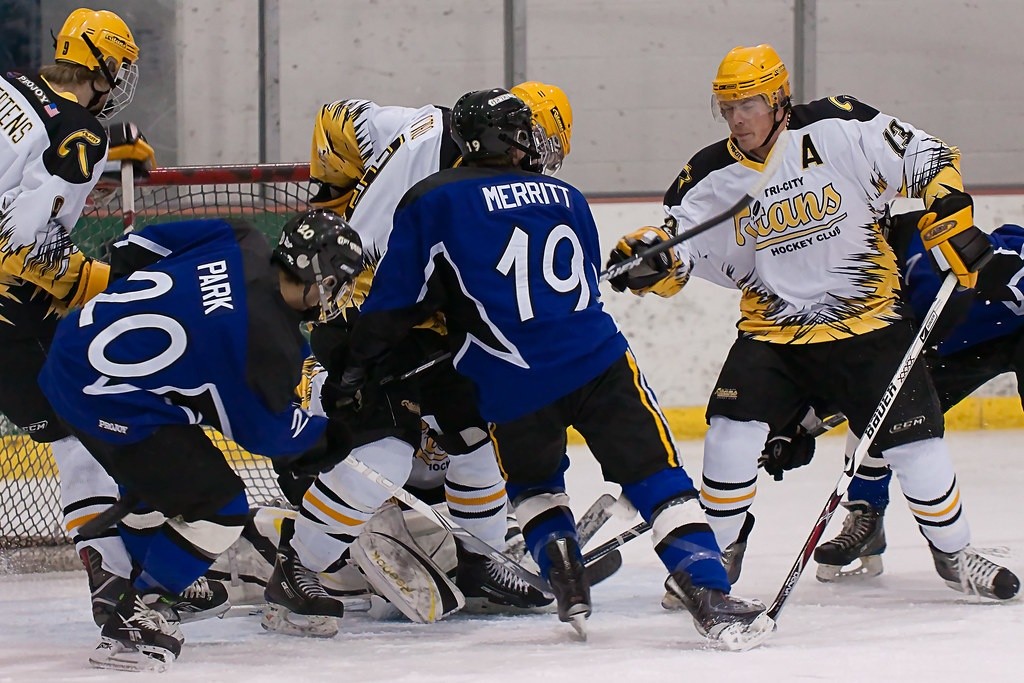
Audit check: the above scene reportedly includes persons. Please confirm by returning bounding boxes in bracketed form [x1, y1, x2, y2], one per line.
[607, 46, 1024, 597]
[812, 221, 1024, 563]
[1, 6, 769, 657]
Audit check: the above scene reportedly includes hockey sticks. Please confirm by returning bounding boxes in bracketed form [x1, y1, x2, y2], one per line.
[117, 156, 136, 238]
[691, 271, 959, 640]
[581, 410, 853, 569]
[342, 454, 622, 600]
[575, 493, 618, 552]
[324, 129, 791, 410]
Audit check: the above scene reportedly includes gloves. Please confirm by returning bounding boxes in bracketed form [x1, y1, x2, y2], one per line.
[762, 423, 817, 482]
[341, 364, 382, 414]
[607, 227, 687, 298]
[919, 194, 995, 291]
[106, 124, 157, 181]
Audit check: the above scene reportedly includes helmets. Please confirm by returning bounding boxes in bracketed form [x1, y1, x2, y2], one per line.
[713, 44, 790, 124]
[513, 80, 573, 175]
[55, 6, 139, 120]
[271, 208, 366, 292]
[450, 88, 536, 161]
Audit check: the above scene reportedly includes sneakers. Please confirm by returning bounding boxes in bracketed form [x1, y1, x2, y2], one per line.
[544, 538, 593, 642]
[665, 573, 778, 653]
[260, 515, 345, 638]
[507, 525, 526, 561]
[92, 587, 179, 672]
[662, 539, 747, 610]
[455, 548, 556, 613]
[932, 544, 1019, 603]
[814, 497, 887, 585]
[77, 541, 230, 625]
